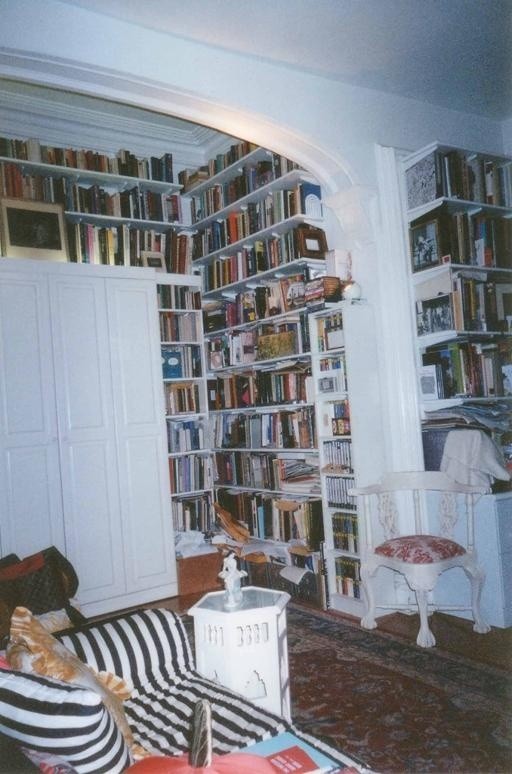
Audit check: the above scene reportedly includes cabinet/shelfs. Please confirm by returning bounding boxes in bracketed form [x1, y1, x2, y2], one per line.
[402, 140, 511, 412]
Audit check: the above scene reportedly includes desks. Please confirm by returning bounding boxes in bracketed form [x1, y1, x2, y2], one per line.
[185, 584, 291, 729]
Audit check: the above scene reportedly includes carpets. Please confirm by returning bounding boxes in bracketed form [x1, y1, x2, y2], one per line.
[171, 600, 512, 774]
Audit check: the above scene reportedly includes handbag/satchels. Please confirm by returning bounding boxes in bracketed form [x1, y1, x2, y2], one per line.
[0, 546, 78, 614]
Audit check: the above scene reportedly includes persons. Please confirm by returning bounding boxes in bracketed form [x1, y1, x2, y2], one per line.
[218, 549, 244, 606]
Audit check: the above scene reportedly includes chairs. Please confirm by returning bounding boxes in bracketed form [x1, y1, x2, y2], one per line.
[346, 471, 491, 647]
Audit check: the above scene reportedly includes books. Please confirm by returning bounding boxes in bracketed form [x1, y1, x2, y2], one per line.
[158, 141, 329, 291]
[214, 451, 322, 495]
[164, 382, 212, 537]
[211, 409, 319, 449]
[318, 355, 362, 600]
[1, 129, 157, 271]
[231, 732, 341, 774]
[214, 487, 325, 603]
[158, 269, 345, 379]
[205, 362, 312, 411]
[404, 148, 512, 497]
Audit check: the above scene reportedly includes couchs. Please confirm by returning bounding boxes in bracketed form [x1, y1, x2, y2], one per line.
[2, 608, 375, 774]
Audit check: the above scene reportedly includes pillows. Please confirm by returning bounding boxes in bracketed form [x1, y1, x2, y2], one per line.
[3, 591, 134, 773]
